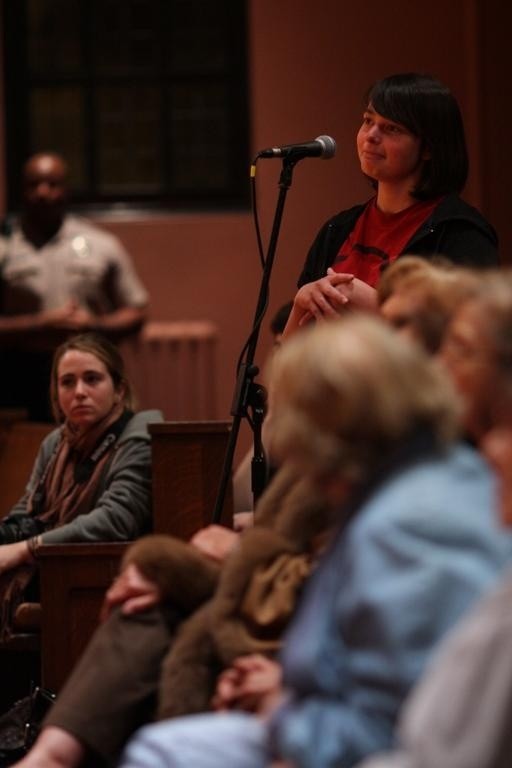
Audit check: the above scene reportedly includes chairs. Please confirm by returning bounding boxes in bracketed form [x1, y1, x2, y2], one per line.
[39, 419, 239, 694]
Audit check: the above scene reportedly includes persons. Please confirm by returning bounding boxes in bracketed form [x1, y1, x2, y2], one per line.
[0, 264, 511, 768]
[276, 69, 501, 349]
[0, 328, 168, 628]
[0, 150, 154, 420]
[13, 256, 511, 767]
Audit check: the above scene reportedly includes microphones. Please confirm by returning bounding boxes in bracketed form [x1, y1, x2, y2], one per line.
[261, 134, 337, 160]
